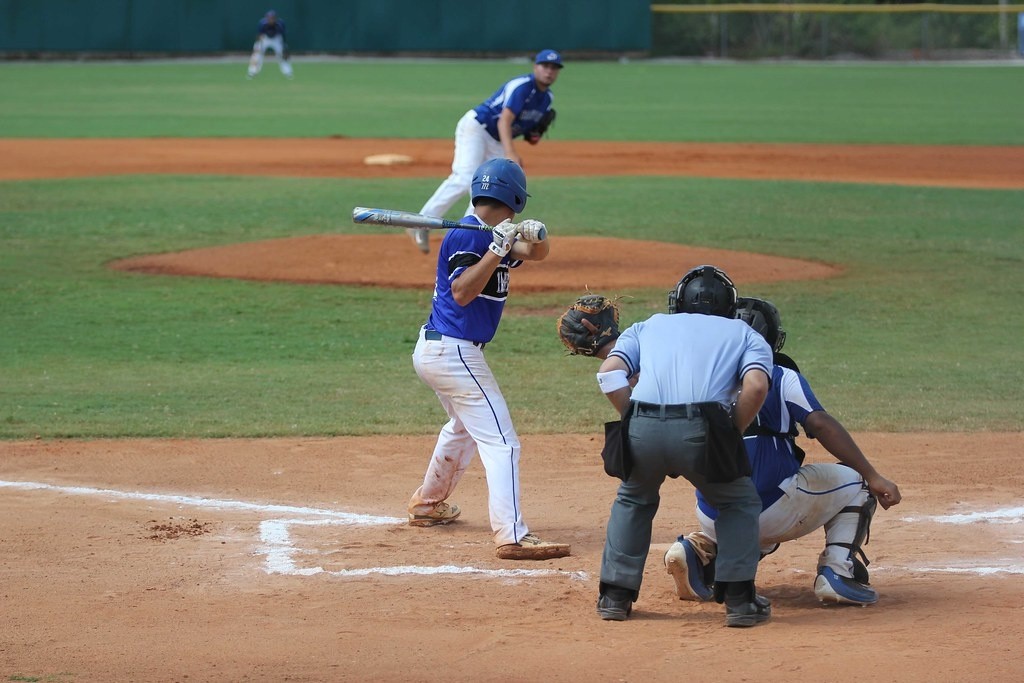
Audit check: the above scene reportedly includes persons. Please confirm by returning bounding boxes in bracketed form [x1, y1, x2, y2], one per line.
[404, 48, 566, 255]
[406, 156, 571, 559]
[561, 263, 902, 628]
[592, 264, 771, 626]
[243, 9, 296, 79]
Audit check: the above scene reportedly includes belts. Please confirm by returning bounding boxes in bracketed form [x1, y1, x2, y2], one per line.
[425, 331, 485, 348]
[476, 116, 500, 142]
[635, 403, 702, 419]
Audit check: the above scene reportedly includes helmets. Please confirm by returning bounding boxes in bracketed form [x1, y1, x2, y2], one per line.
[736, 297, 787, 353]
[471, 158, 531, 213]
[667, 265, 737, 319]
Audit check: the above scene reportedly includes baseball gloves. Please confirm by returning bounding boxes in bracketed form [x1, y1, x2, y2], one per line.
[523, 108, 556, 145]
[556, 284, 636, 358]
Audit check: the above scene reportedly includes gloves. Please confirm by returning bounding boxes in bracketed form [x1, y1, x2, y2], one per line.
[516, 219, 547, 243]
[489, 218, 519, 256]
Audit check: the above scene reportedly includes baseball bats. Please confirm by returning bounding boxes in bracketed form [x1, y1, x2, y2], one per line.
[351, 205, 548, 242]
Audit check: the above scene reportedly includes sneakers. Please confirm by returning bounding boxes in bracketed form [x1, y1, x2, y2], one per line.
[407, 227, 430, 254]
[812, 566, 879, 608]
[409, 504, 461, 527]
[596, 581, 634, 619]
[496, 534, 571, 560]
[664, 535, 714, 602]
[714, 581, 772, 625]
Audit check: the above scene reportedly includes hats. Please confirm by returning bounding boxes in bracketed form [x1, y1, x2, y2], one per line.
[536, 50, 563, 70]
